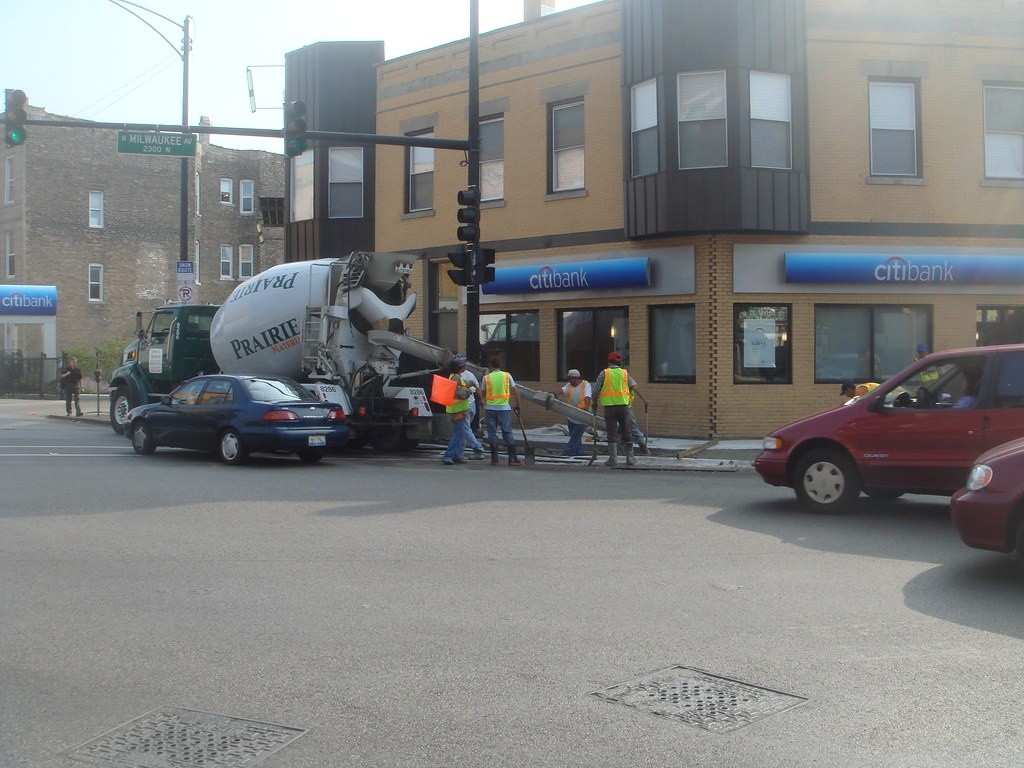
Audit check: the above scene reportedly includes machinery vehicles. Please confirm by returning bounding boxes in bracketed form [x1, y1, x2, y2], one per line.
[106, 250, 622, 491]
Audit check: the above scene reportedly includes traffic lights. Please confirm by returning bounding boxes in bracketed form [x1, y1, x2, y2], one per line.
[446, 250, 472, 286]
[455, 188, 481, 242]
[3, 88, 28, 147]
[475, 246, 496, 284]
[283, 100, 309, 157]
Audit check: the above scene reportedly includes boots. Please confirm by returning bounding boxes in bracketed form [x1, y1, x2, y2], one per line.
[638, 440, 649, 454]
[489, 443, 498, 465]
[605, 443, 618, 466]
[468, 451, 485, 460]
[507, 442, 521, 466]
[626, 442, 637, 464]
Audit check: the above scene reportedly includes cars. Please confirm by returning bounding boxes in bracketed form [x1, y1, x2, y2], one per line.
[948, 436, 1024, 562]
[121, 374, 351, 467]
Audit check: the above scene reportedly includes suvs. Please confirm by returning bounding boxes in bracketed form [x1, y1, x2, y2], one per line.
[753, 342, 1024, 514]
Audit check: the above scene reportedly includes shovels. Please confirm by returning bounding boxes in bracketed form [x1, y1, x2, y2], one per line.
[588, 405, 599, 466]
[513, 406, 536, 466]
[642, 403, 651, 455]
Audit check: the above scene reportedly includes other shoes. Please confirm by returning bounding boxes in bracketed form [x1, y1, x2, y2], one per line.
[444, 456, 454, 465]
[457, 458, 468, 463]
[67, 412, 72, 416]
[77, 413, 83, 416]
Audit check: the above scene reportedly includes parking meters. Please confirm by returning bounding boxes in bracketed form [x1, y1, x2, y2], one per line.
[94, 368, 102, 416]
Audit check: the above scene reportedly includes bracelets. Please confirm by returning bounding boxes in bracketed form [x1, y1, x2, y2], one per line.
[82, 387, 84, 389]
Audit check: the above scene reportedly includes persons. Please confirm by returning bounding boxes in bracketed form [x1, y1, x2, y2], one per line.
[912, 343, 945, 382]
[442, 353, 484, 465]
[591, 352, 650, 468]
[952, 370, 986, 410]
[840, 382, 881, 398]
[554, 369, 592, 456]
[61, 357, 85, 417]
[481, 356, 523, 466]
[1, 358, 23, 399]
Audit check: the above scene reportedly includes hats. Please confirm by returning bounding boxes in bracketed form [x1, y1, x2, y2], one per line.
[917, 343, 928, 352]
[608, 352, 622, 362]
[568, 369, 580, 377]
[456, 358, 467, 367]
[840, 382, 854, 395]
